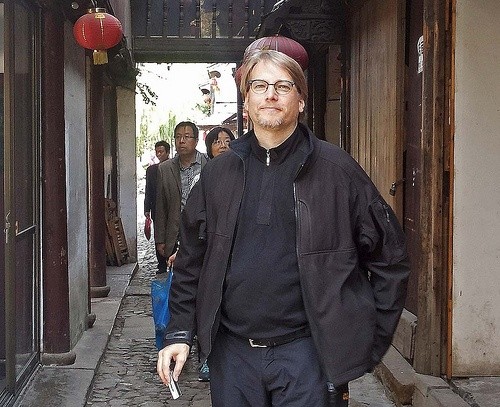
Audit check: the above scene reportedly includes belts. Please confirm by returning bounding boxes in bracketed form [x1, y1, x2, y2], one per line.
[218, 321, 313, 348]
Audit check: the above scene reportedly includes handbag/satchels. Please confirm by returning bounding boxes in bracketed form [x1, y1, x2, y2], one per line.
[151, 271, 173, 349]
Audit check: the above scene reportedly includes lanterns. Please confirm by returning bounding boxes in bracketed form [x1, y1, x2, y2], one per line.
[235, 65, 250, 92]
[73, 8, 122, 66]
[245, 36, 309, 72]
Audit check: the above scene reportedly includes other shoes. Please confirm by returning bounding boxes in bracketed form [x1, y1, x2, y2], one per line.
[156, 268, 167, 274]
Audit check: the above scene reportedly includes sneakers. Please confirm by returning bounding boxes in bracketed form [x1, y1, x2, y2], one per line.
[198, 361, 211, 382]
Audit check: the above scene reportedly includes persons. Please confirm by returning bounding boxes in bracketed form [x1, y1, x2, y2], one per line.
[156, 50, 406, 407]
[169, 127, 235, 268]
[144, 140, 170, 273]
[156, 121, 211, 381]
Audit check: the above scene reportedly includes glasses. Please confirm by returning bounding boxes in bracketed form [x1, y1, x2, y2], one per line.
[174, 133, 195, 141]
[212, 138, 231, 148]
[247, 78, 296, 95]
